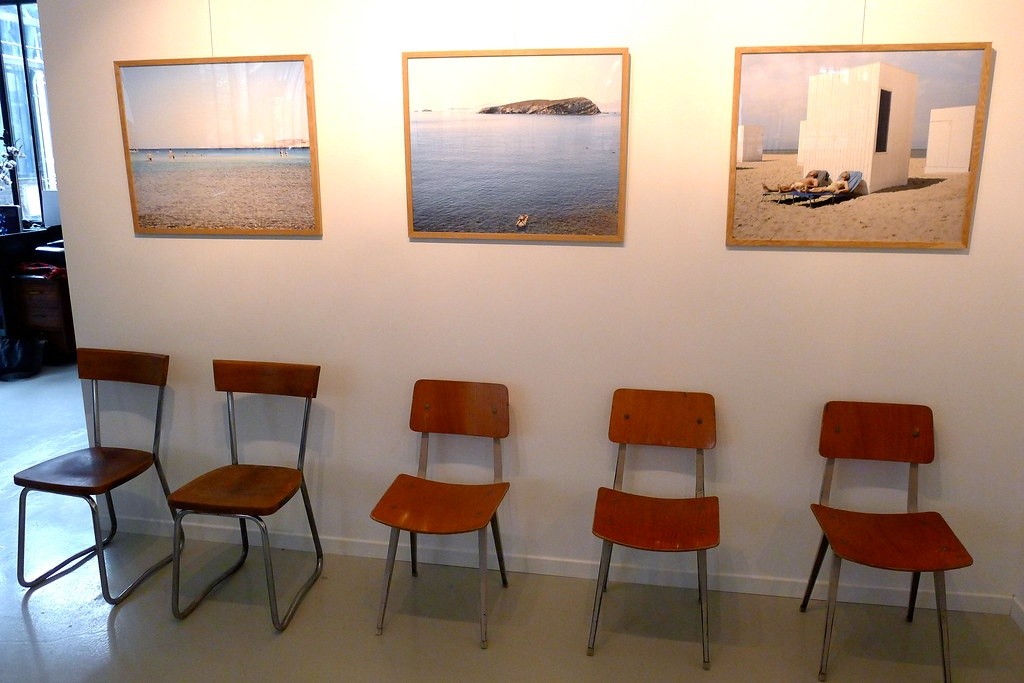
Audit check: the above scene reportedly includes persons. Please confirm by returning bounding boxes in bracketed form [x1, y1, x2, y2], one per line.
[762, 170, 850, 195]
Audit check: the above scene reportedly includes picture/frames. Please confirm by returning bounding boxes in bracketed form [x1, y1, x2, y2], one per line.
[727, 43, 990, 254]
[395, 49, 630, 246]
[111, 55, 325, 237]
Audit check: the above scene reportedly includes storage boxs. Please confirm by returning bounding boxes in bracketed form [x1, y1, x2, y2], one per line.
[13, 277, 73, 348]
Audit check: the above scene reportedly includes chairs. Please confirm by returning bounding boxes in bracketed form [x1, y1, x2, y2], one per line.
[584, 386, 723, 668]
[166, 358, 326, 633]
[761, 169, 863, 207]
[799, 398, 972, 683]
[12, 345, 188, 604]
[370, 377, 511, 649]
